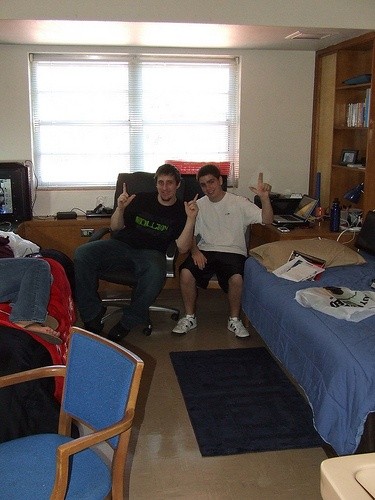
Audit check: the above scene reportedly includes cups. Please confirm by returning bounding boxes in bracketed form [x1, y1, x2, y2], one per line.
[315, 207, 325, 217]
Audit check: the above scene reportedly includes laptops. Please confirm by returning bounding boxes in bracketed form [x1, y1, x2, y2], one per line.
[275, 195, 319, 223]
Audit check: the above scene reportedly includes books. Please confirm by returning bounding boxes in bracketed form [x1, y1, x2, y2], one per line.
[347, 88, 371, 127]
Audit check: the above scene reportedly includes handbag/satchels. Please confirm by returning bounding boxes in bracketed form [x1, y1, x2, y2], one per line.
[293, 286, 375, 323]
[249, 239, 367, 273]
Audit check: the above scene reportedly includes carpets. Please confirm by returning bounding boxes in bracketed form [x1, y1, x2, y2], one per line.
[170, 348, 327, 458]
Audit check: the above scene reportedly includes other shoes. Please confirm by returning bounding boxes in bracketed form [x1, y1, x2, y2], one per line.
[83, 304, 130, 342]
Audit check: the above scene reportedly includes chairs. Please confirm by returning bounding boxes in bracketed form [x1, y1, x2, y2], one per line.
[0, 327, 144, 500]
[176, 173, 250, 280]
[88, 171, 182, 335]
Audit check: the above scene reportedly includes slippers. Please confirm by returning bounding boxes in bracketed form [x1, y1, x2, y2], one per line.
[11, 315, 63, 345]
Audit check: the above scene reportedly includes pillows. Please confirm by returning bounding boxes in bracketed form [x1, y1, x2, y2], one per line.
[250, 241, 366, 271]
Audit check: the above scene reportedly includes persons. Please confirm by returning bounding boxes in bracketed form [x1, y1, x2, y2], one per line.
[0, 258, 63, 345]
[172, 165, 274, 337]
[74, 163, 199, 344]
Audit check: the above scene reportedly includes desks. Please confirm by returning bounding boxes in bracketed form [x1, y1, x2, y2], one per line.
[251, 214, 358, 251]
[320, 453, 375, 500]
[24, 216, 183, 291]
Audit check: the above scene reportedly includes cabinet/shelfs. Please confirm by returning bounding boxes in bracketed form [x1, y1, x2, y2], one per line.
[309, 30, 375, 224]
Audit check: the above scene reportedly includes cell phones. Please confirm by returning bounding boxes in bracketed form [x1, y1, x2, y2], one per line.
[278, 227, 290, 232]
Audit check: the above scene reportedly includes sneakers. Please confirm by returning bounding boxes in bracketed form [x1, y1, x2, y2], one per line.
[172, 314, 198, 334]
[226, 317, 250, 338]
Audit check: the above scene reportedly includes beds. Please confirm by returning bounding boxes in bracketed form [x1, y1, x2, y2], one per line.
[243, 239, 375, 456]
[0, 229, 77, 437]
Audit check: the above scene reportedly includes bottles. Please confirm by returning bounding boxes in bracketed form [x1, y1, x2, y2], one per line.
[330, 201, 339, 232]
